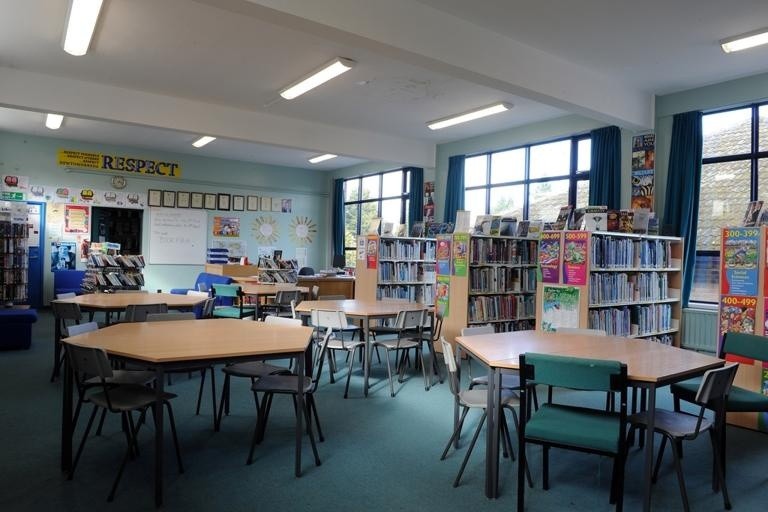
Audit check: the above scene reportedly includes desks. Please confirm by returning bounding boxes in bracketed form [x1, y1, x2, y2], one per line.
[63, 318, 315, 510]
[454, 331, 731, 511]
[296, 298, 432, 398]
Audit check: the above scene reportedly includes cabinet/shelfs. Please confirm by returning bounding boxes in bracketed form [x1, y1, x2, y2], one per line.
[714, 223, 767, 437]
[536, 229, 685, 348]
[427, 233, 538, 362]
[1, 197, 32, 306]
[349, 234, 434, 336]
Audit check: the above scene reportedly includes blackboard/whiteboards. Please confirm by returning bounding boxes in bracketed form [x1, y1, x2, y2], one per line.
[91, 206, 144, 255]
[149, 206, 207, 264]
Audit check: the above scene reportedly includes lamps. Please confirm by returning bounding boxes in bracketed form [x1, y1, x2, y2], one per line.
[58, 0, 101, 60]
[190, 135, 216, 149]
[307, 153, 337, 165]
[273, 54, 356, 103]
[41, 112, 63, 130]
[426, 102, 513, 132]
[719, 27, 767, 59]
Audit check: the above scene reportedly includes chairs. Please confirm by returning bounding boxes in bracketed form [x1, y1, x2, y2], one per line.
[452, 325, 543, 456]
[51, 264, 443, 339]
[217, 316, 302, 432]
[0, 304, 38, 349]
[671, 332, 767, 478]
[51, 301, 85, 386]
[66, 319, 158, 437]
[289, 298, 300, 370]
[146, 311, 219, 430]
[309, 308, 364, 400]
[438, 337, 534, 491]
[517, 352, 629, 511]
[61, 340, 183, 502]
[630, 364, 738, 511]
[245, 327, 333, 479]
[368, 309, 430, 397]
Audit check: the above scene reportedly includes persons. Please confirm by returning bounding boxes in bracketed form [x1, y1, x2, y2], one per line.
[51, 246, 75, 270]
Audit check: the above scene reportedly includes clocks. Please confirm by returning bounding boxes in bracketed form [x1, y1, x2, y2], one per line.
[111, 175, 127, 190]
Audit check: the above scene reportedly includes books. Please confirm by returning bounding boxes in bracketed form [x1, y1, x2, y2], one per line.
[81, 253, 146, 293]
[1, 221, 30, 303]
[370, 218, 455, 306]
[588, 235, 672, 347]
[552, 205, 659, 235]
[258, 258, 298, 285]
[468, 215, 542, 332]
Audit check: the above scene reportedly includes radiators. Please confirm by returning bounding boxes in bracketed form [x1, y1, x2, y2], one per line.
[682, 308, 719, 354]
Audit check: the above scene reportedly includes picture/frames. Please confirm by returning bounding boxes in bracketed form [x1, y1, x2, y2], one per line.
[146, 190, 293, 212]
